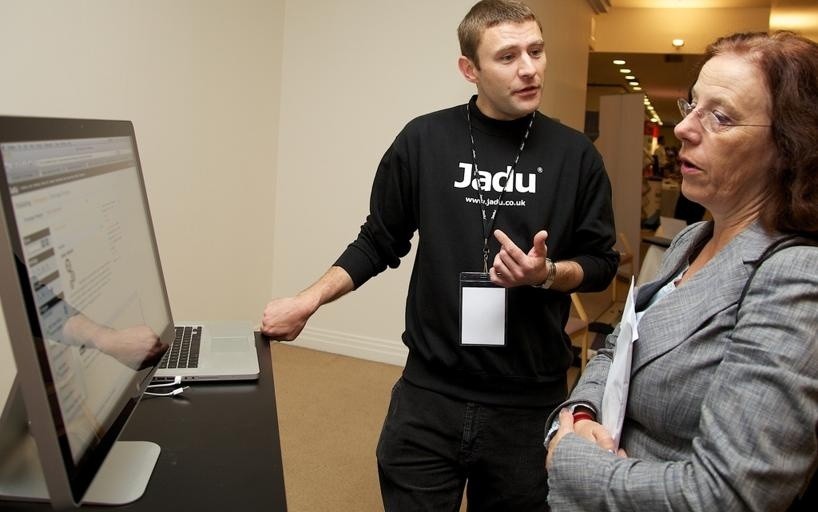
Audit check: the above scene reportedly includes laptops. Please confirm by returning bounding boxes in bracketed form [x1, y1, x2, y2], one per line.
[151, 318, 260, 384]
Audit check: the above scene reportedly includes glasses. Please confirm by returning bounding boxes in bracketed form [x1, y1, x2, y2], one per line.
[676, 96, 772, 135]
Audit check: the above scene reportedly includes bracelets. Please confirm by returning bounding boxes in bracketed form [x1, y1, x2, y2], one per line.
[530, 257, 557, 291]
[570, 414, 594, 424]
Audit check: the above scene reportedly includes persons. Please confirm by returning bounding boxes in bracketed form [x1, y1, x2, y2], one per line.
[654, 135, 675, 174]
[258, 0, 623, 512]
[26, 269, 172, 374]
[539, 24, 817, 511]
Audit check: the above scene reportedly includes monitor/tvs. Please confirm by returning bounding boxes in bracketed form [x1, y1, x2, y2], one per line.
[0, 115, 178, 510]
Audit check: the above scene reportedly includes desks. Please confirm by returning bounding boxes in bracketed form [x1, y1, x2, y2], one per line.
[0, 331, 288, 512]
